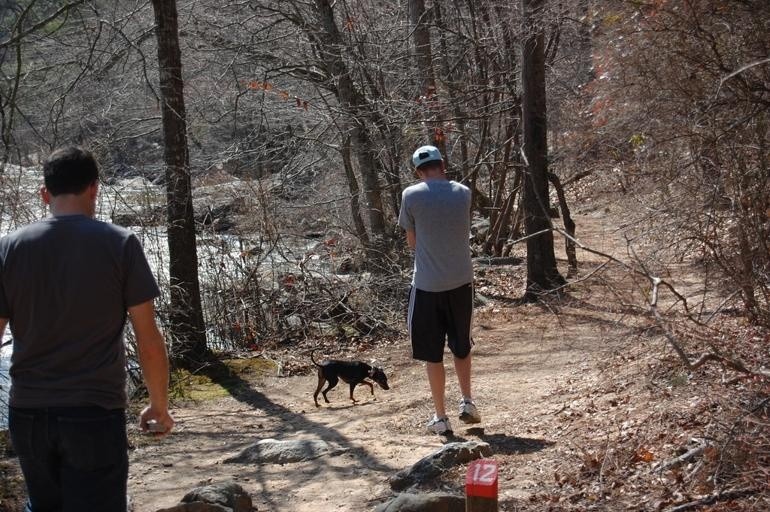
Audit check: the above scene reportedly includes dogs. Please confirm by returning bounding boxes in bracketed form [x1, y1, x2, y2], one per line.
[311, 347, 389, 408]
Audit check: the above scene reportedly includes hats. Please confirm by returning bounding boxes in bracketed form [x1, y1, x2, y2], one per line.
[410, 145, 443, 173]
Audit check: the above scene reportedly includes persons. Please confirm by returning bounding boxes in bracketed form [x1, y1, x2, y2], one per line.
[1, 145, 174, 512]
[397, 145, 481, 436]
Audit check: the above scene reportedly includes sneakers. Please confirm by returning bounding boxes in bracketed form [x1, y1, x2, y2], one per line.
[459, 395, 481, 423]
[427, 418, 453, 435]
